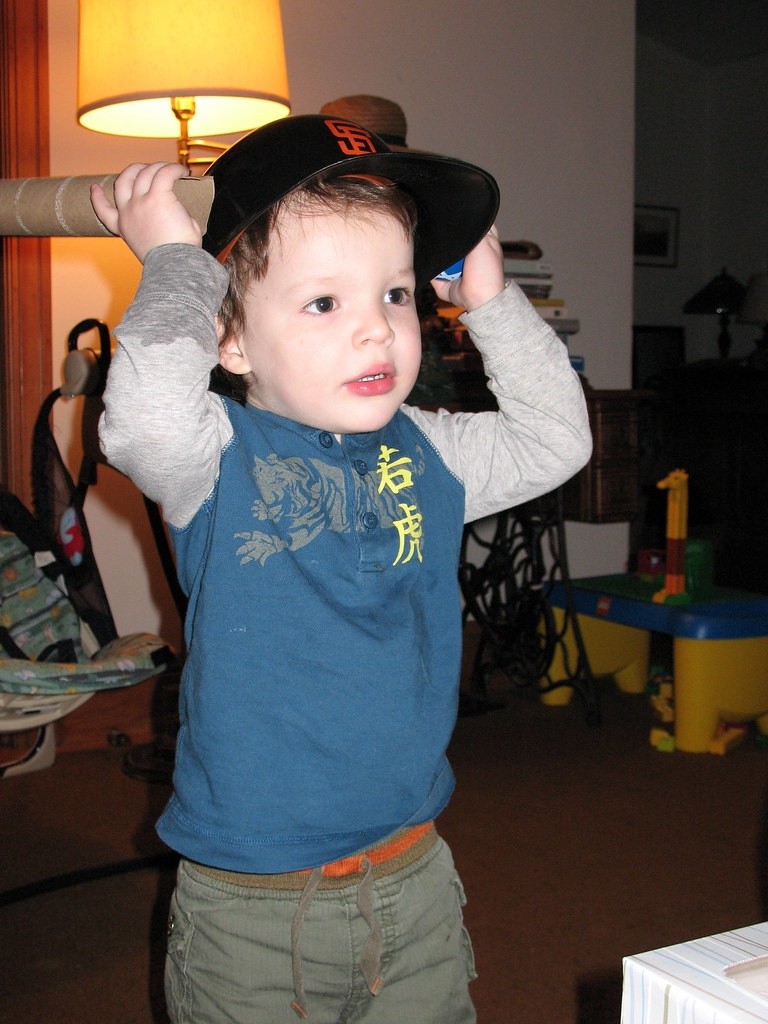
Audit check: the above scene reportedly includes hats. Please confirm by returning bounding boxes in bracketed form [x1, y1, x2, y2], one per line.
[318, 94, 424, 154]
[202, 113, 501, 292]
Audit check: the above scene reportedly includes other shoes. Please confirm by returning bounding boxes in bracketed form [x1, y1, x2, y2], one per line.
[122, 735, 176, 782]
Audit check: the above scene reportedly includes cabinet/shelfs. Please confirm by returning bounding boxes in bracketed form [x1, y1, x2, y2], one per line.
[536, 389, 666, 562]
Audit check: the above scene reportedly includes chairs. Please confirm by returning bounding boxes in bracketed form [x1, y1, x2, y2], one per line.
[0, 493, 177, 775]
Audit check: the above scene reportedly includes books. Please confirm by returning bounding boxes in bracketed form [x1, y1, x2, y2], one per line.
[502, 258, 585, 373]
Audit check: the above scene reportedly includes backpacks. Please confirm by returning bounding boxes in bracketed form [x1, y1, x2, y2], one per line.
[34, 316, 199, 662]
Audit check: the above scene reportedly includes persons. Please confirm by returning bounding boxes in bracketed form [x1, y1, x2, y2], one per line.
[89, 113, 594, 1024]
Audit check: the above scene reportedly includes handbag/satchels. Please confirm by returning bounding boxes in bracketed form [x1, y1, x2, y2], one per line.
[0, 491, 179, 696]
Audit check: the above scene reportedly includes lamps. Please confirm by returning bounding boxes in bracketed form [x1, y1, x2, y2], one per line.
[683, 266, 747, 359]
[734, 273, 768, 369]
[78, 0, 291, 178]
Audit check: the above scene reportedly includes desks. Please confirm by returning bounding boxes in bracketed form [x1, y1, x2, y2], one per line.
[645, 361, 768, 595]
[538, 573, 768, 753]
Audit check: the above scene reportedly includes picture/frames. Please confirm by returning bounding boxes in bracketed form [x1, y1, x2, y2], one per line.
[632, 325, 685, 390]
[633, 205, 681, 268]
